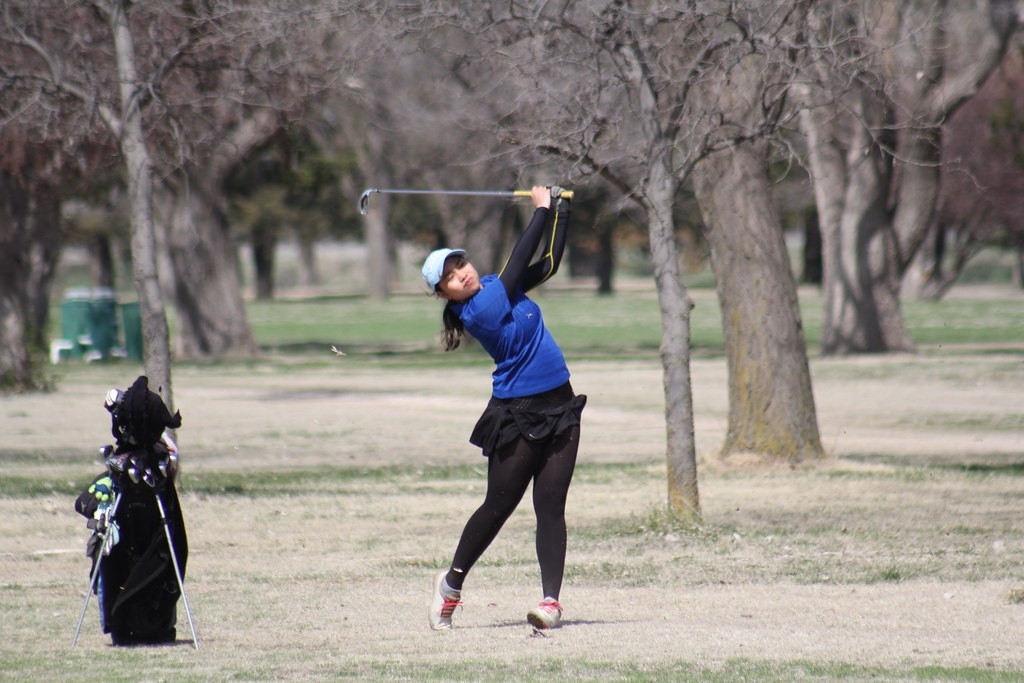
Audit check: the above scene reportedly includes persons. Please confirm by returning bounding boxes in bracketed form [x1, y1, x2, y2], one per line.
[422, 184, 589, 629]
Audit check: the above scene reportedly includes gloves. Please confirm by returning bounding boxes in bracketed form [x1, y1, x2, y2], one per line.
[546, 183, 571, 212]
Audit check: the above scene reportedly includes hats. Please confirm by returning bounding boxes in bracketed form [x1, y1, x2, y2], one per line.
[422, 247, 466, 292]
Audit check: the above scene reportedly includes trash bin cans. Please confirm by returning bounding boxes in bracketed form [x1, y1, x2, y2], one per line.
[45, 289, 146, 359]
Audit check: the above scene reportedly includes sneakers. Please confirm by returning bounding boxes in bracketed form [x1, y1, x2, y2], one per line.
[527, 595, 563, 630]
[428, 569, 461, 630]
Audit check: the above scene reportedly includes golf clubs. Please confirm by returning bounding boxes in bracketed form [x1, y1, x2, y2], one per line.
[108, 451, 178, 488]
[357, 188, 574, 214]
